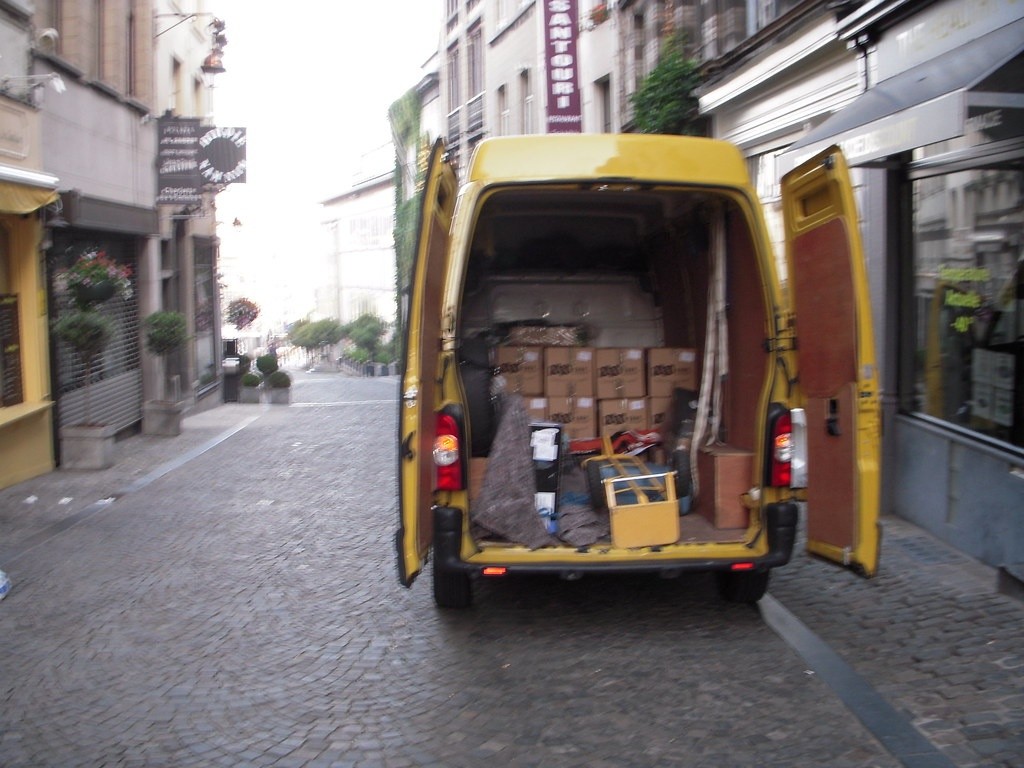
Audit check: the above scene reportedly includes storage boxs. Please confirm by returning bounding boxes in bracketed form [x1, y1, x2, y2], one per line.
[649, 401, 674, 435]
[492, 345, 544, 395]
[648, 349, 703, 399]
[522, 399, 546, 424]
[600, 401, 646, 441]
[550, 400, 598, 440]
[597, 349, 648, 399]
[546, 348, 597, 397]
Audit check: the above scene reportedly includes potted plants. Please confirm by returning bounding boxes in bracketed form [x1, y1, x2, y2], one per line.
[239, 374, 261, 403]
[142, 310, 190, 436]
[265, 371, 291, 403]
[50, 314, 118, 472]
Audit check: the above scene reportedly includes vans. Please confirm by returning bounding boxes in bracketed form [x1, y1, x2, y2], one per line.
[391, 131, 885, 612]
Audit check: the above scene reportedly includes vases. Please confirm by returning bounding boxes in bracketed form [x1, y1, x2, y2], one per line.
[73, 284, 114, 302]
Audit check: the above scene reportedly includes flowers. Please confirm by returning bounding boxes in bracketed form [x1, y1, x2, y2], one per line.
[55, 249, 136, 300]
[228, 299, 258, 329]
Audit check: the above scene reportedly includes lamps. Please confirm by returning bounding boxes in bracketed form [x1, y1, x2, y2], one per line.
[154, 9, 227, 73]
[1, 71, 66, 94]
